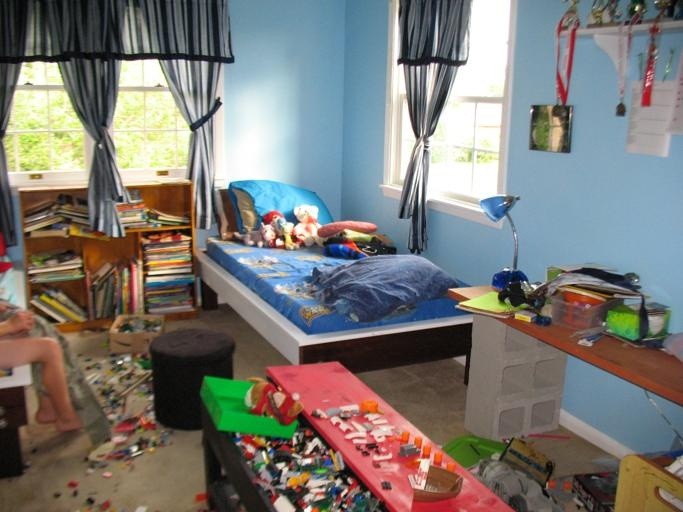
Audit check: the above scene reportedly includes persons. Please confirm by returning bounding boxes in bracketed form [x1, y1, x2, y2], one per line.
[0, 301, 100, 433]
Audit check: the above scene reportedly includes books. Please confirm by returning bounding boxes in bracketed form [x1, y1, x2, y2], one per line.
[22, 195, 195, 323]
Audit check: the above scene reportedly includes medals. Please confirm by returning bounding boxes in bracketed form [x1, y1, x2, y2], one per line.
[616, 103, 626, 116]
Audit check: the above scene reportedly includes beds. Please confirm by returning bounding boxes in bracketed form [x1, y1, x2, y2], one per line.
[192, 234, 476, 384]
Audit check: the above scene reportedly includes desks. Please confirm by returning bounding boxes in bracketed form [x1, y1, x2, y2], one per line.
[17, 180, 201, 333]
[447, 285, 683, 440]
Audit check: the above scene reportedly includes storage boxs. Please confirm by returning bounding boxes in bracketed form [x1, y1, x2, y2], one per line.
[612, 450, 683, 512]
[110, 313, 165, 352]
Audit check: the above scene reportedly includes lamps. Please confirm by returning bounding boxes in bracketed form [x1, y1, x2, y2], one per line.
[481, 194, 528, 290]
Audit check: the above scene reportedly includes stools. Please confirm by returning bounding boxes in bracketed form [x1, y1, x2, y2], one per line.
[0, 363, 41, 474]
[149, 328, 237, 431]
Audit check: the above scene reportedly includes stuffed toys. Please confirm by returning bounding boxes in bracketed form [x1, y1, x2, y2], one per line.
[244, 377, 304, 425]
[233, 204, 324, 250]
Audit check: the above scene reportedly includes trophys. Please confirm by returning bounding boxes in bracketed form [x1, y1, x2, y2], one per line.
[558, 0, 673, 30]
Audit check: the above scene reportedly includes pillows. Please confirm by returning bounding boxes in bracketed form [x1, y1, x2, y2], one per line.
[216, 189, 239, 240]
[227, 179, 334, 234]
